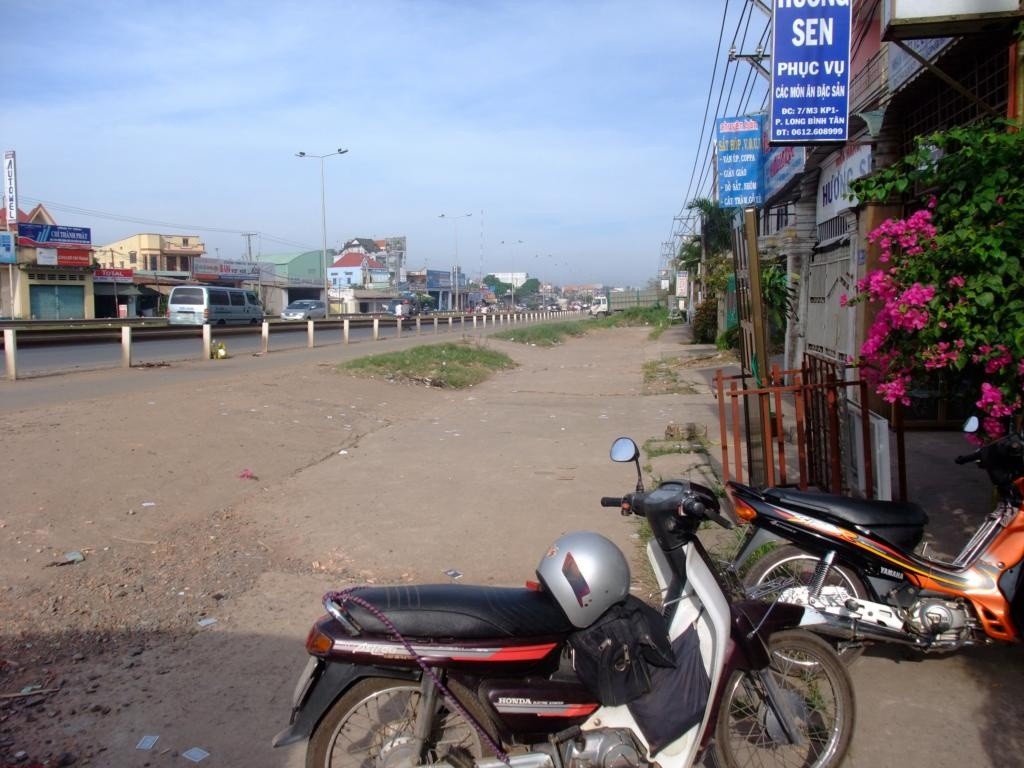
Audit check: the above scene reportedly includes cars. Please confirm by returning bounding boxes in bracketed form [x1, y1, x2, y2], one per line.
[386, 299, 420, 317]
[280, 299, 330, 322]
[546, 303, 561, 312]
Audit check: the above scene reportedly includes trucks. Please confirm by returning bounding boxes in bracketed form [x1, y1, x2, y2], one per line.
[589, 288, 668, 320]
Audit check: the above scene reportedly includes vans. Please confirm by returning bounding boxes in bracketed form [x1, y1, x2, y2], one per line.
[164, 284, 264, 328]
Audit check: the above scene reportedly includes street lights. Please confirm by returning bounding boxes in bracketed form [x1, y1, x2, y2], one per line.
[438, 213, 473, 311]
[501, 240, 524, 310]
[295, 147, 349, 319]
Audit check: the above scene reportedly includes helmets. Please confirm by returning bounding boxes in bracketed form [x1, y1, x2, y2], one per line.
[535, 532, 631, 629]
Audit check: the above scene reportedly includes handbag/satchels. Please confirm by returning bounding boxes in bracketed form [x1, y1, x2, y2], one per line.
[561, 595, 678, 707]
[628, 619, 711, 758]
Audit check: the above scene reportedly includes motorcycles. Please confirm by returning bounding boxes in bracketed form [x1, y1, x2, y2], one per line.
[267, 434, 860, 768]
[718, 415, 1024, 680]
